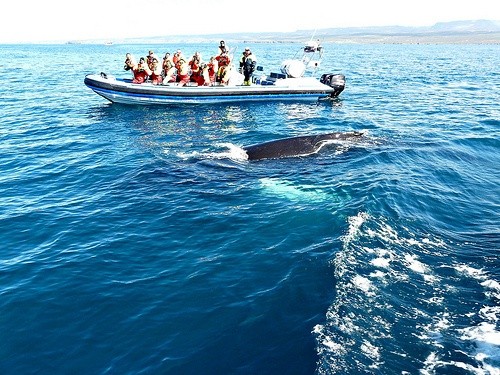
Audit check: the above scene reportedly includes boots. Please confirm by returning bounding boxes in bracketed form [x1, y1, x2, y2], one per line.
[248, 80, 250, 86]
[244, 81, 247, 86]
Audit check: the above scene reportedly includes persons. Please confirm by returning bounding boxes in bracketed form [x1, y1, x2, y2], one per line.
[125, 50, 230, 87]
[240, 48, 256, 87]
[218, 41, 229, 54]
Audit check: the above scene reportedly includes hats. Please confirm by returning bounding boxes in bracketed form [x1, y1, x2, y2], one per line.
[245, 47, 250, 51]
[149, 50, 153, 54]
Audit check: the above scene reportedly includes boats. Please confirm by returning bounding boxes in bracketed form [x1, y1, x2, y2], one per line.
[83, 31, 346, 109]
